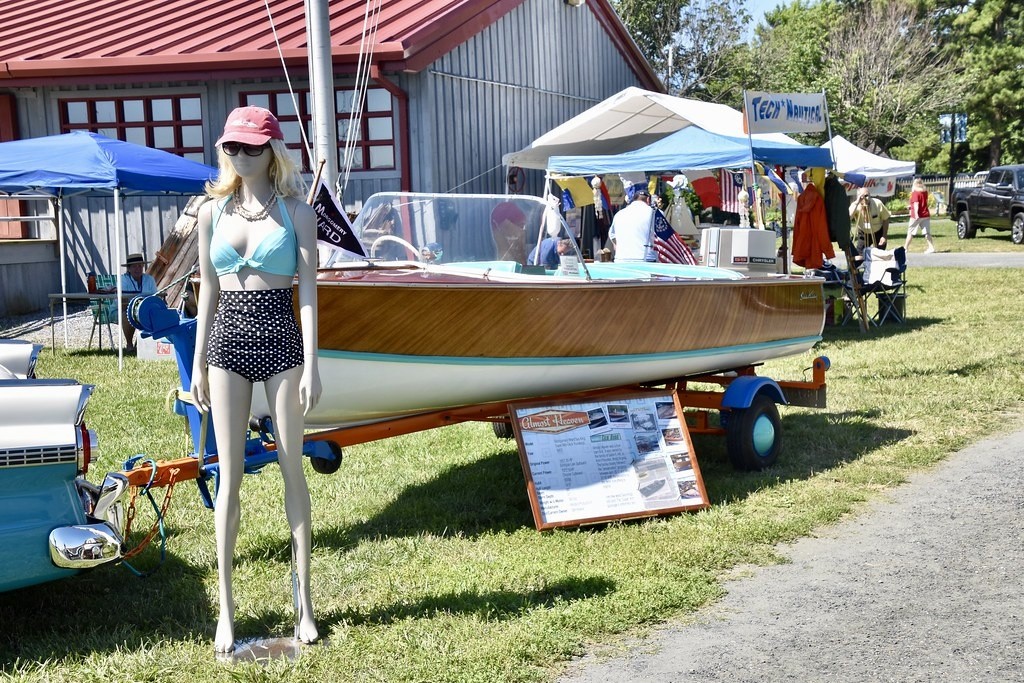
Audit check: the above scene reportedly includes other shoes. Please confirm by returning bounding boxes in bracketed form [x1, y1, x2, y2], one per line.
[127, 344, 135, 353]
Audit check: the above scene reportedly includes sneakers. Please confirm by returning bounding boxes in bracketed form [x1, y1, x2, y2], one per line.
[924, 247, 935, 254]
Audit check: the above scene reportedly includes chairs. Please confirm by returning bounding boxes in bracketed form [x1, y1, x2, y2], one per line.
[86, 274, 118, 351]
[837, 246, 908, 329]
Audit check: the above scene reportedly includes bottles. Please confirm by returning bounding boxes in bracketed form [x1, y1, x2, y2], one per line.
[87, 273, 96, 294]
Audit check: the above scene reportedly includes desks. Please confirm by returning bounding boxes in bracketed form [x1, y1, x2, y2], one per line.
[47, 292, 168, 356]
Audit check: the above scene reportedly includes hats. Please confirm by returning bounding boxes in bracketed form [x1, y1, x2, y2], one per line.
[121, 254, 151, 266]
[635, 189, 651, 197]
[215, 105, 284, 148]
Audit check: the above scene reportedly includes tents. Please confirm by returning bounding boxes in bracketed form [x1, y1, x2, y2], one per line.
[0, 130, 221, 369]
[544, 124, 833, 273]
[820, 135, 916, 182]
[501, 86, 807, 196]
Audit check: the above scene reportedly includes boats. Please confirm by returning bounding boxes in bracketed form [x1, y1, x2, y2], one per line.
[131, 1, 830, 457]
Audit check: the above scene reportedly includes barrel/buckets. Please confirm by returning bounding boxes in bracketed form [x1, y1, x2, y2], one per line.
[877, 293, 905, 320]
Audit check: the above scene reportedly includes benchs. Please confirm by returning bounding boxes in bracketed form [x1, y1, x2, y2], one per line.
[446, 261, 747, 280]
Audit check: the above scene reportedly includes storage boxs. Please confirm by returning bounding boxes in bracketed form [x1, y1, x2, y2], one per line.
[822, 281, 843, 299]
[136, 331, 176, 361]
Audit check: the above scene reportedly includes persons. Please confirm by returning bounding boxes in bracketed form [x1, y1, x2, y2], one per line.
[524, 236, 573, 270]
[191, 104, 323, 652]
[904, 178, 937, 254]
[606, 190, 655, 262]
[849, 188, 891, 252]
[120, 254, 157, 352]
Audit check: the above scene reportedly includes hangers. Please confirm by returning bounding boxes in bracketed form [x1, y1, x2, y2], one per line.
[808, 174, 814, 184]
[829, 170, 835, 178]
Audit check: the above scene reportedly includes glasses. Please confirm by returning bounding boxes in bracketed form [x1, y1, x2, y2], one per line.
[222, 141, 271, 156]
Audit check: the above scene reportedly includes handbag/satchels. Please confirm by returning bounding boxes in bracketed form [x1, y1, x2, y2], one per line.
[825, 295, 844, 325]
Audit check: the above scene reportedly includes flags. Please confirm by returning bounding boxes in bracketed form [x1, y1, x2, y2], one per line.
[618, 172, 648, 199]
[649, 209, 699, 266]
[755, 161, 793, 196]
[720, 168, 745, 213]
[680, 168, 721, 208]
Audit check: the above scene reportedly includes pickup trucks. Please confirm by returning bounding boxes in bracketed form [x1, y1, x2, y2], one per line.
[949, 165, 1024, 244]
